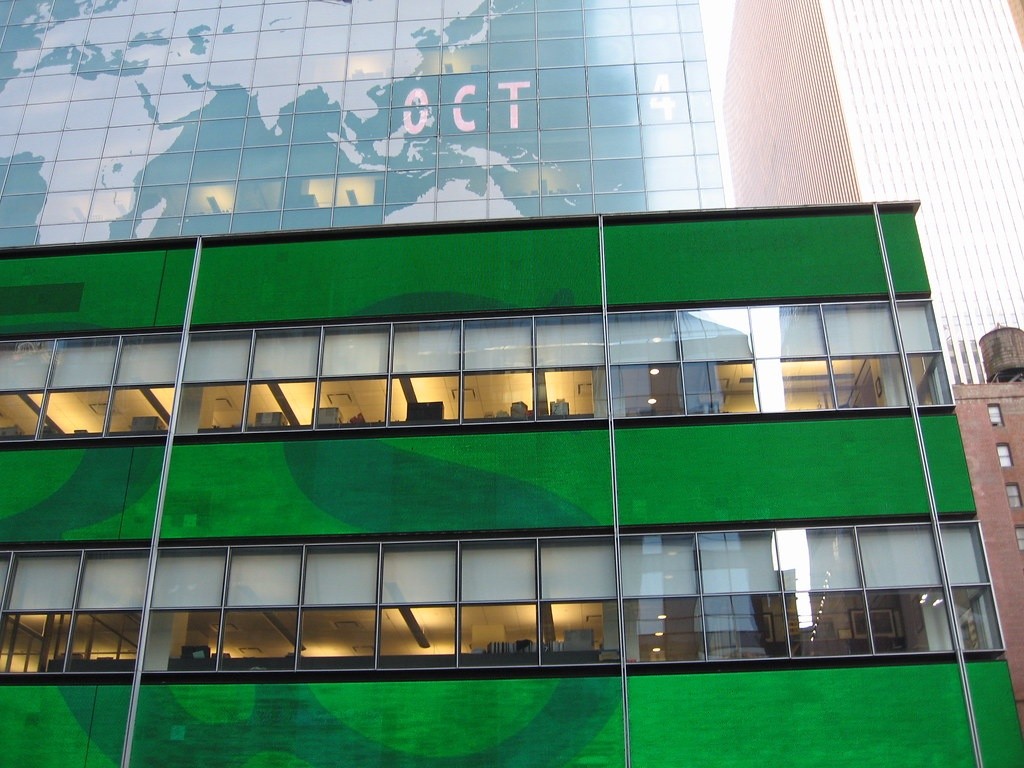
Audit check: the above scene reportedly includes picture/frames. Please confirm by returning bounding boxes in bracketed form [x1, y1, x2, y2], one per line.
[912, 594, 924, 634]
[848, 608, 897, 640]
[875, 377, 882, 398]
[762, 612, 775, 644]
